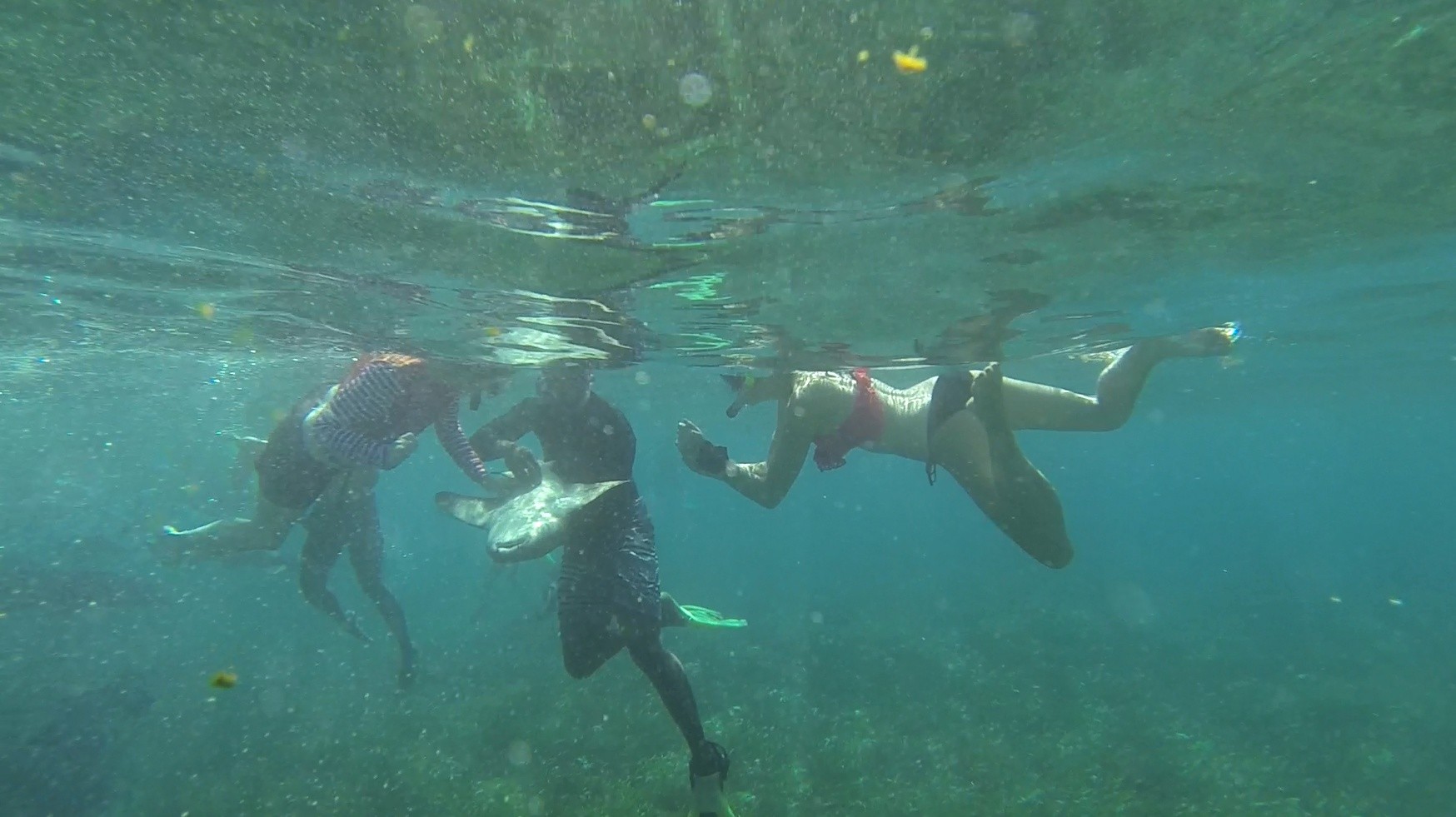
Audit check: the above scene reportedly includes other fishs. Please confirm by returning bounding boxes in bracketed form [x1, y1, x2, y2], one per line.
[432, 459, 633, 564]
[206, 670, 237, 690]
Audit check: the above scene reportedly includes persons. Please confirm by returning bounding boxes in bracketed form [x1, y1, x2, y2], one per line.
[150, 347, 521, 562]
[293, 465, 423, 699]
[675, 324, 1233, 570]
[467, 356, 733, 817]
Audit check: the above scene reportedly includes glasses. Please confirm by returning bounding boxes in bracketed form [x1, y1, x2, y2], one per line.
[547, 370, 598, 390]
